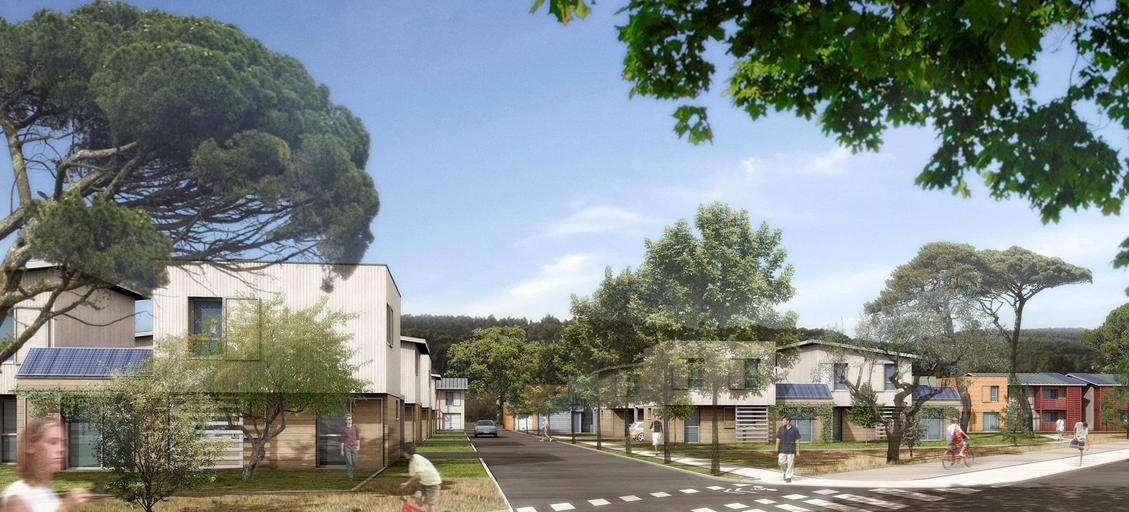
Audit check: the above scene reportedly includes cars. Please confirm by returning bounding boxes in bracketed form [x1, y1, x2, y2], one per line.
[629, 422, 644, 442]
[474, 420, 497, 438]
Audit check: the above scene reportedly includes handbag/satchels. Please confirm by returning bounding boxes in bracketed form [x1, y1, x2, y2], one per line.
[953, 424, 963, 443]
[651, 422, 655, 431]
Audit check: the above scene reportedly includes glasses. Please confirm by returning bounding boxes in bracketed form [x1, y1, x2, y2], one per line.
[348, 418, 352, 420]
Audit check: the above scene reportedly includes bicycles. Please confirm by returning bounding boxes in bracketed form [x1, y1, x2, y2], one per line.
[942, 439, 974, 469]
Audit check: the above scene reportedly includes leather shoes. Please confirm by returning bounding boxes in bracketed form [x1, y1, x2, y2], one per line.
[783, 474, 791, 483]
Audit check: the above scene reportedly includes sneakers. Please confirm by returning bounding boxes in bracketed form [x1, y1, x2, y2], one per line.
[540, 438, 553, 442]
